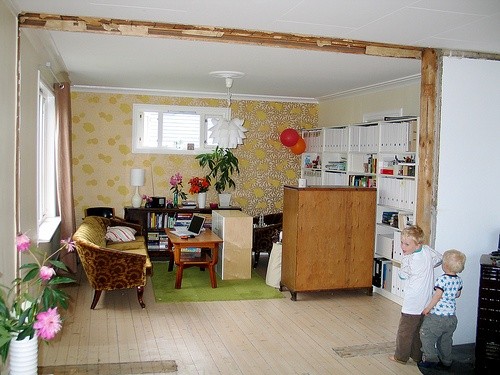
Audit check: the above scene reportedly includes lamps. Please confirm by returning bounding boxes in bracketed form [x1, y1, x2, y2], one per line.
[130, 168, 145, 207]
[207, 71, 249, 148]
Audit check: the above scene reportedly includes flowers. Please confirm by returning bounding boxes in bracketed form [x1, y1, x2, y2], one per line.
[169, 173, 188, 200]
[187, 176, 211, 195]
[0, 231, 77, 364]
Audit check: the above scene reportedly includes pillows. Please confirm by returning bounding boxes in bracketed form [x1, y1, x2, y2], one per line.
[104, 225, 138, 242]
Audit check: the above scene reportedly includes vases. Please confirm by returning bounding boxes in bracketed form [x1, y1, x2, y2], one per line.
[10, 330, 38, 375]
[198, 193, 205, 208]
[174, 186, 178, 205]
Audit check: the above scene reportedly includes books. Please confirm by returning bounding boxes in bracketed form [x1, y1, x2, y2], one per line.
[305, 153, 415, 187]
[380, 210, 419, 233]
[147, 210, 211, 254]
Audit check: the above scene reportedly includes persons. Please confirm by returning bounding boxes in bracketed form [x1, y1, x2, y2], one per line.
[415, 249, 467, 366]
[389, 226, 443, 363]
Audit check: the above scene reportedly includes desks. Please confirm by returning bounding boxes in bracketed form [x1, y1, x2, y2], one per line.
[163, 227, 223, 290]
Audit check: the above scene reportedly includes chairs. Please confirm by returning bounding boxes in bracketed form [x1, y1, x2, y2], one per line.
[252, 213, 283, 264]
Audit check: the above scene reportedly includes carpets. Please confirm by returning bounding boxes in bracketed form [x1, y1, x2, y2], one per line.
[151, 262, 287, 304]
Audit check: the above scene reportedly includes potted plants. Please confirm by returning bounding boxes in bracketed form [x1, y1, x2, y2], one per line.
[196, 150, 239, 206]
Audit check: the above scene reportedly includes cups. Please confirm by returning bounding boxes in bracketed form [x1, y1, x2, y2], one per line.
[299, 179, 307, 188]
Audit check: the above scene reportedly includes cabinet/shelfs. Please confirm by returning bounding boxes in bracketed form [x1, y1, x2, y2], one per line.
[279, 184, 376, 301]
[473, 264, 500, 375]
[124, 206, 243, 262]
[210, 209, 253, 280]
[300, 114, 420, 305]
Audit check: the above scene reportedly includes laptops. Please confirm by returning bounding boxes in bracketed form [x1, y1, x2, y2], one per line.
[170, 213, 206, 236]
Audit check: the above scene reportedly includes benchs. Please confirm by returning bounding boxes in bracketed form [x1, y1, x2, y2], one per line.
[72, 215, 154, 310]
